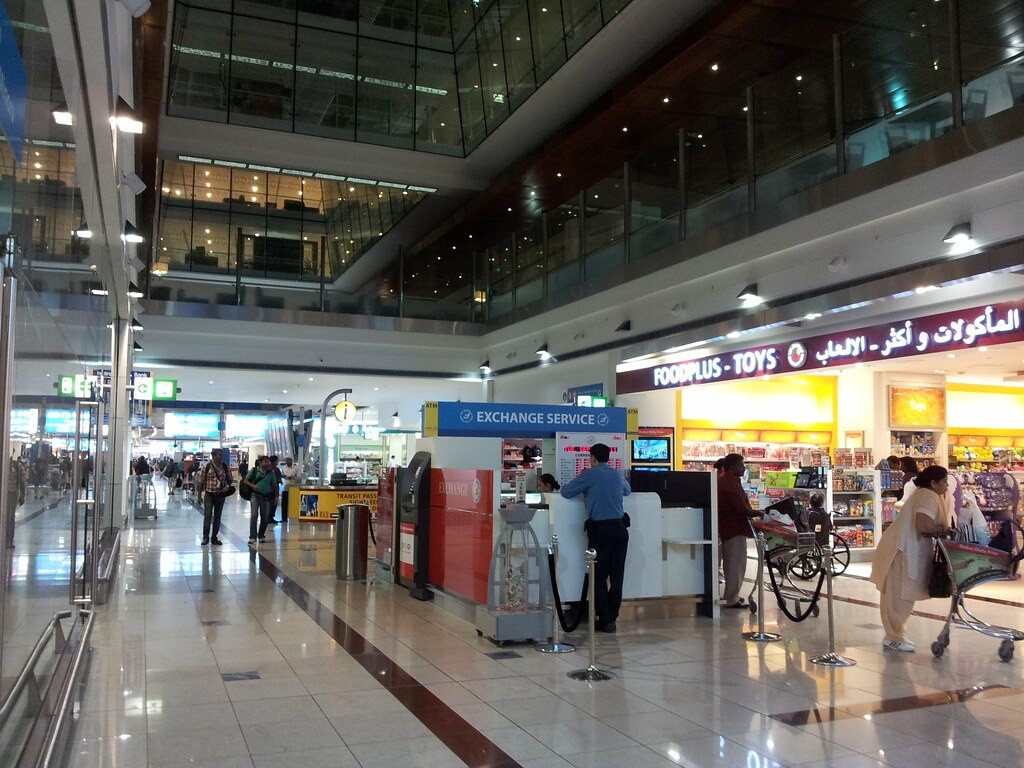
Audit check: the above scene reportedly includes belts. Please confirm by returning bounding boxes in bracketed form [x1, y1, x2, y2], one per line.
[593, 518, 625, 527]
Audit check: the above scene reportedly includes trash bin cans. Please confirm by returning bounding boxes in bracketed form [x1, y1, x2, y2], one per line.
[336, 504, 369, 580]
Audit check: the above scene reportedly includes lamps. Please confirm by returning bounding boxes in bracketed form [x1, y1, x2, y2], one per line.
[506, 332, 582, 361]
[151, 262, 168, 281]
[92, 282, 108, 296]
[134, 341, 144, 352]
[116, 94, 143, 135]
[51, 100, 72, 125]
[133, 317, 144, 331]
[76, 222, 92, 238]
[131, 302, 146, 314]
[614, 319, 630, 332]
[826, 256, 845, 273]
[121, 169, 146, 195]
[670, 303, 686, 316]
[127, 255, 146, 273]
[736, 282, 757, 299]
[129, 281, 143, 298]
[317, 360, 490, 418]
[475, 290, 487, 302]
[125, 219, 143, 243]
[942, 223, 971, 244]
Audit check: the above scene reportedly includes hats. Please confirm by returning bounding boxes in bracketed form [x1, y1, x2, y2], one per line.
[285, 455, 294, 460]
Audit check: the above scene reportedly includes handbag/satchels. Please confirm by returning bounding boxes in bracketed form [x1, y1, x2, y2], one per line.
[175, 475, 182, 488]
[927, 542, 951, 598]
[239, 479, 253, 501]
[221, 485, 236, 497]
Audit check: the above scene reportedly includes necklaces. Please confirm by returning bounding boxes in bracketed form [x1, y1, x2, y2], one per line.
[244, 456, 277, 544]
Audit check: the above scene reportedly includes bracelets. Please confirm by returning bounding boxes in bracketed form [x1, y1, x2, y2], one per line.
[225, 484, 229, 488]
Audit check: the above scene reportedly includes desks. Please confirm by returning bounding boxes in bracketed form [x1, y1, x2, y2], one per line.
[150, 286, 172, 301]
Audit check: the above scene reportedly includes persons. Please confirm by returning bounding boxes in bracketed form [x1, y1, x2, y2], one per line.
[537, 474, 561, 493]
[713, 458, 726, 582]
[355, 456, 360, 462]
[882, 455, 902, 497]
[239, 459, 248, 479]
[868, 465, 951, 653]
[270, 455, 283, 524]
[159, 458, 181, 495]
[279, 457, 298, 522]
[898, 456, 919, 500]
[388, 455, 395, 466]
[560, 443, 632, 633]
[806, 493, 833, 575]
[718, 453, 764, 609]
[197, 449, 233, 544]
[314, 456, 319, 482]
[135, 456, 150, 475]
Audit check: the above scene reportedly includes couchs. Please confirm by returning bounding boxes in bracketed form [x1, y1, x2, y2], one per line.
[184, 195, 320, 267]
[176, 289, 209, 304]
[215, 286, 400, 318]
[1, 174, 67, 187]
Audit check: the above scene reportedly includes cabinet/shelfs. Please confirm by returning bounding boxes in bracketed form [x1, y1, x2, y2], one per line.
[682, 438, 1024, 564]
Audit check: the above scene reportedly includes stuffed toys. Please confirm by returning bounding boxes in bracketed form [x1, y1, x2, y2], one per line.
[953, 445, 1024, 461]
[956, 462, 1024, 473]
[953, 472, 989, 507]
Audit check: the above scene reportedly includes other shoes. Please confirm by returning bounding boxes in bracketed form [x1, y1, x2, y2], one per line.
[248, 538, 257, 544]
[727, 602, 749, 609]
[267, 518, 278, 524]
[736, 596, 744, 603]
[201, 540, 208, 545]
[168, 492, 174, 496]
[280, 520, 287, 522]
[211, 540, 222, 545]
[259, 538, 265, 543]
[595, 622, 616, 633]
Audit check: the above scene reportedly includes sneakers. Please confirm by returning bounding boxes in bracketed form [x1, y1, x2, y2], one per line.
[903, 638, 914, 645]
[882, 639, 915, 651]
